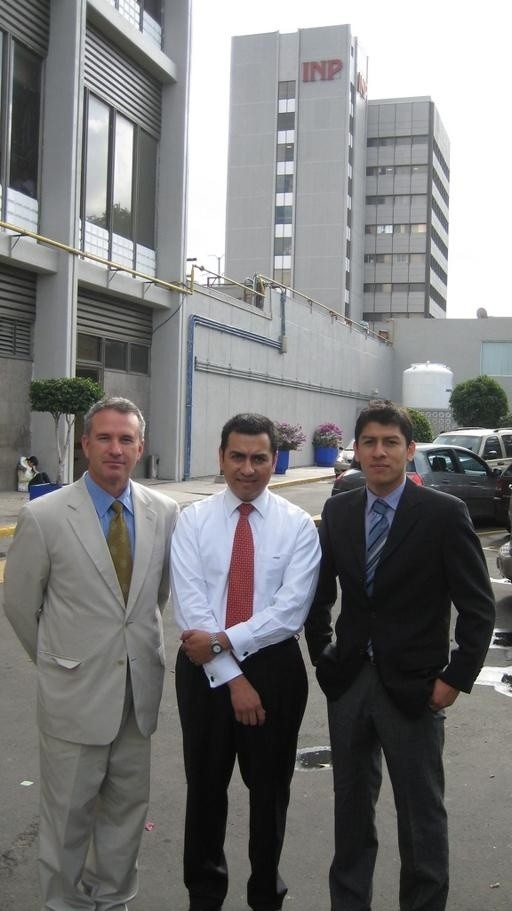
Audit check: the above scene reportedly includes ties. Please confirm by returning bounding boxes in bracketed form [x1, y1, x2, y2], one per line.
[226, 502, 254, 628]
[106, 499, 133, 607]
[365, 499, 391, 659]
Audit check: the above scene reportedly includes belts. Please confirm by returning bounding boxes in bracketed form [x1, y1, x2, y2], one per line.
[361, 655, 380, 666]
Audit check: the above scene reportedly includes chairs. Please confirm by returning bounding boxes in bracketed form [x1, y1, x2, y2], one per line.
[432, 457, 450, 472]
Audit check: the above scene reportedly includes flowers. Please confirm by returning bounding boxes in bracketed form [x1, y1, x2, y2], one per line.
[274, 420, 306, 451]
[312, 423, 343, 448]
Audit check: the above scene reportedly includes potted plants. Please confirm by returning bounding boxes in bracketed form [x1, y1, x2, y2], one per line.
[29, 375, 105, 501]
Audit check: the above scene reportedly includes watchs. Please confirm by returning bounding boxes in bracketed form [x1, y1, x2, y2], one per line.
[208, 633, 224, 656]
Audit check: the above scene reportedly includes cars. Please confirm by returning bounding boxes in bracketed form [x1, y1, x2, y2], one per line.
[496, 539, 511, 586]
[328, 424, 512, 533]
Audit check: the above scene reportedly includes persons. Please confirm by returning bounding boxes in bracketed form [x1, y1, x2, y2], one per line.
[165, 408, 327, 911]
[298, 402, 500, 911]
[17, 455, 52, 493]
[1, 394, 183, 911]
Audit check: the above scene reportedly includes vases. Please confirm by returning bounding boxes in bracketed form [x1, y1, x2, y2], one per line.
[275, 448, 289, 474]
[314, 446, 339, 467]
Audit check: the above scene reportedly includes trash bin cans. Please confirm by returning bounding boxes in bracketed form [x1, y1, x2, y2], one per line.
[146, 455, 159, 478]
